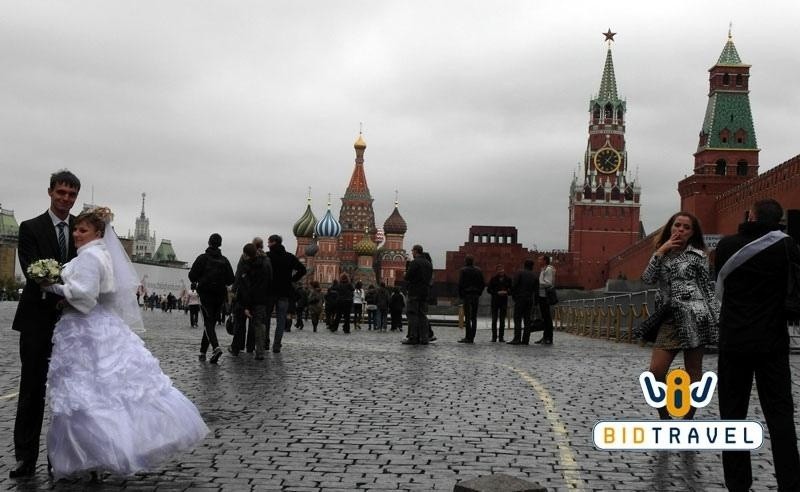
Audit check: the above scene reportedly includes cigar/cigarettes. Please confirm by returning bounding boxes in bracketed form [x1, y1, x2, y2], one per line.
[672, 234, 680, 236]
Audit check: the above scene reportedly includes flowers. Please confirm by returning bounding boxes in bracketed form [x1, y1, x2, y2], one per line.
[27, 258, 65, 299]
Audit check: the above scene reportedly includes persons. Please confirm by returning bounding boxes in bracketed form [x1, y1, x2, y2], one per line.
[141, 232, 559, 362]
[715, 199, 799, 492]
[10, 171, 80, 478]
[642, 212, 719, 419]
[39, 208, 211, 482]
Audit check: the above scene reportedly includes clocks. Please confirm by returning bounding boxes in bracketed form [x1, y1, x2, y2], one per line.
[594, 146, 621, 175]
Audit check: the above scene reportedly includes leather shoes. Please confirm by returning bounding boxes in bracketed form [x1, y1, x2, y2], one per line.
[401, 334, 438, 344]
[489, 339, 497, 342]
[499, 339, 505, 342]
[534, 338, 553, 344]
[506, 339, 521, 344]
[457, 338, 474, 343]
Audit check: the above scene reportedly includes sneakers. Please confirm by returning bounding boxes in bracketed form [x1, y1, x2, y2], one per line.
[8, 460, 37, 476]
[227, 343, 281, 360]
[210, 349, 223, 363]
[199, 352, 206, 362]
